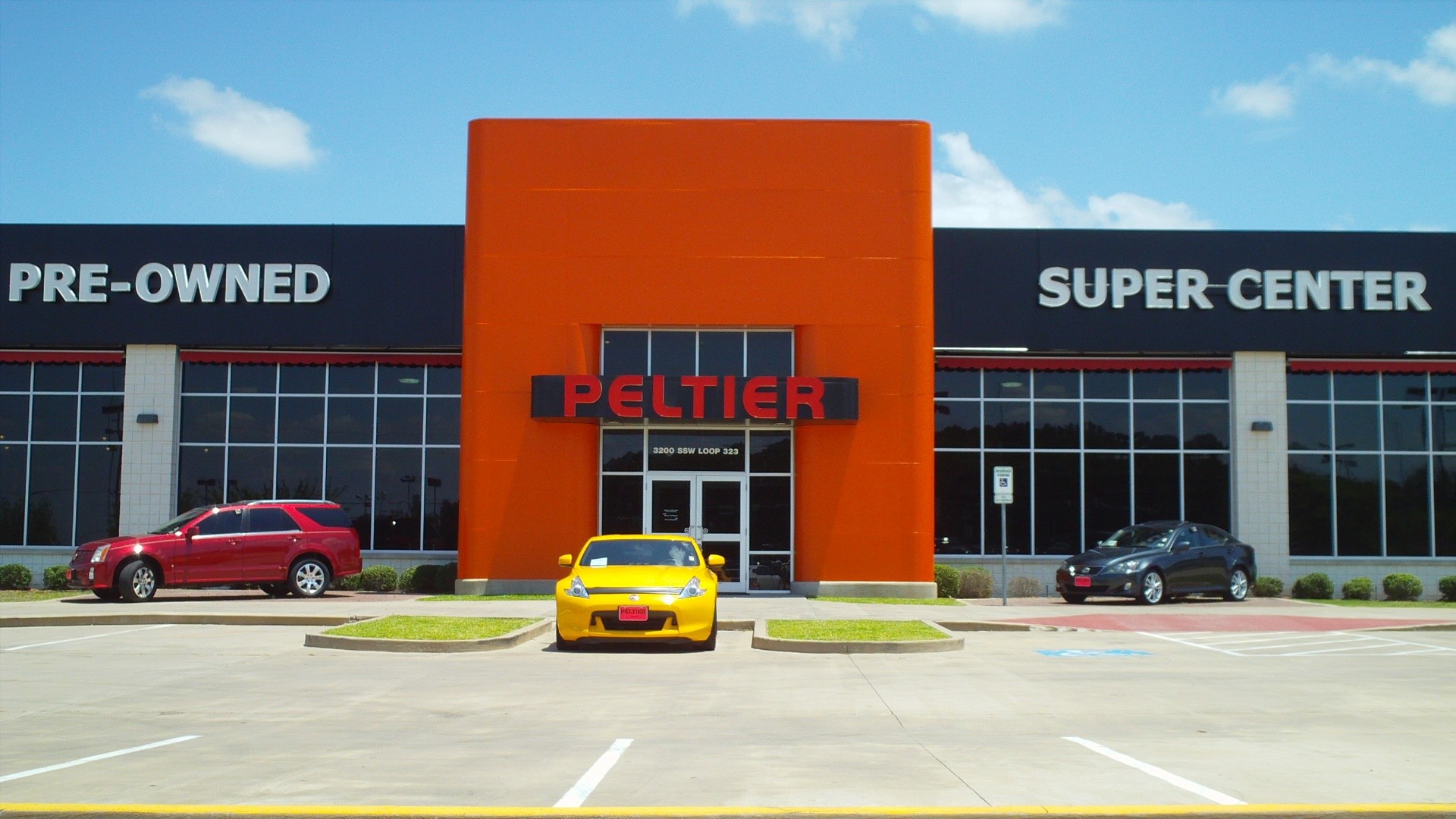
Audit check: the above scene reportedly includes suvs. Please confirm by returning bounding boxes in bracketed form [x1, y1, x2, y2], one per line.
[64, 499, 363, 603]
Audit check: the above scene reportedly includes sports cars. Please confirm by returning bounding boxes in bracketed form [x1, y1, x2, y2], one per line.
[555, 533, 726, 651]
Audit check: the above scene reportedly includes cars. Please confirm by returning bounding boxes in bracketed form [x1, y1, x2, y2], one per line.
[1055, 521, 1255, 605]
